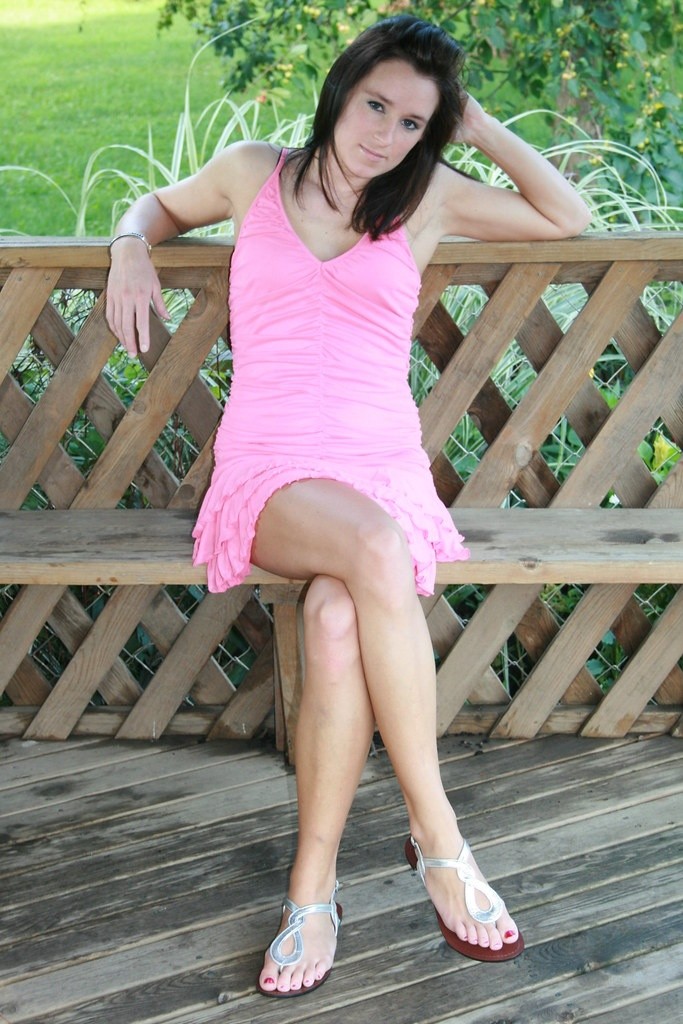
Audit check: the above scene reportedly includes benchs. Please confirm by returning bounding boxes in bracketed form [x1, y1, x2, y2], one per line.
[3, 510, 682, 769]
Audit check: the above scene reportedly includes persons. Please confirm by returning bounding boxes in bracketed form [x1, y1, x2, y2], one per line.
[99, 20, 592, 1001]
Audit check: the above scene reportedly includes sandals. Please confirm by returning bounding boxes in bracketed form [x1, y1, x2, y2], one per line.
[404, 835, 524, 963]
[255, 878, 344, 998]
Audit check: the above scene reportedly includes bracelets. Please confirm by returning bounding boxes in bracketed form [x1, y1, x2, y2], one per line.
[106, 233, 154, 263]
